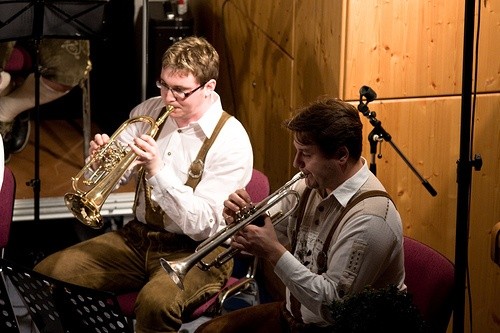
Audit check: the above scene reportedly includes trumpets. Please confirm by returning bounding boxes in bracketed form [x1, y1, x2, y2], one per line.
[63, 104, 177, 231]
[158, 171, 306, 295]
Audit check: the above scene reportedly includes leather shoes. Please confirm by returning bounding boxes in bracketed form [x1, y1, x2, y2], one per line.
[0, 119, 31, 164]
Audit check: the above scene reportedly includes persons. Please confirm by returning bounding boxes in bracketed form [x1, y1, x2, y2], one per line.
[0, 0, 94, 164]
[33, 36, 254, 333]
[194, 93, 408, 333]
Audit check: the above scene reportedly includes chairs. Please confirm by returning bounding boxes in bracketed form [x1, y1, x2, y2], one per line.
[106, 168, 270, 333]
[404, 235, 456, 333]
[4, 47, 91, 166]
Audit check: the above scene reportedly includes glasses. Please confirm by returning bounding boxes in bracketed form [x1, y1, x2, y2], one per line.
[156, 79, 208, 100]
